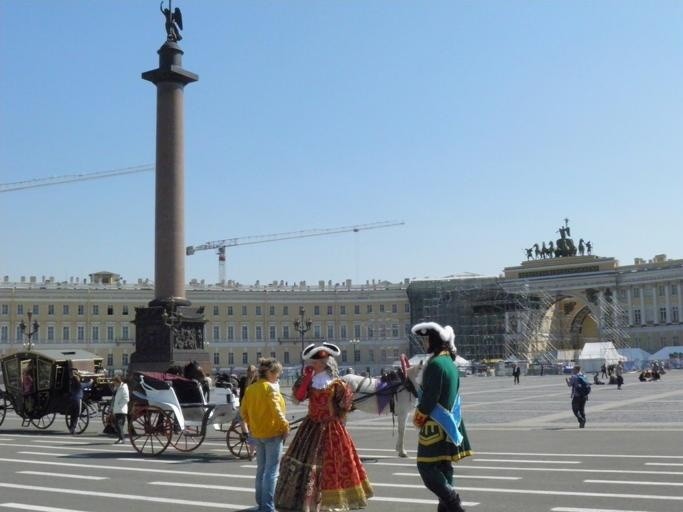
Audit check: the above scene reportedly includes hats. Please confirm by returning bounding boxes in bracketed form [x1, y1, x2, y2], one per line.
[411, 320, 456, 354]
[301, 342, 340, 362]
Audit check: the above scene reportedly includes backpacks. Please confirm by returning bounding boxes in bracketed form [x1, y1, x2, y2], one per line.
[574, 373, 591, 397]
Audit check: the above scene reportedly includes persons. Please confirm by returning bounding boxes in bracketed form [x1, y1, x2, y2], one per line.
[202, 374, 212, 403]
[238, 365, 261, 442]
[273, 341, 373, 512]
[238, 358, 290, 512]
[592, 359, 667, 391]
[159, 0, 184, 41]
[409, 321, 474, 512]
[565, 366, 589, 429]
[66, 372, 94, 435]
[511, 363, 520, 384]
[111, 375, 130, 443]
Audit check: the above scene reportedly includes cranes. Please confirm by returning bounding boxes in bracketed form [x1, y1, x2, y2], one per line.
[187, 219, 404, 278]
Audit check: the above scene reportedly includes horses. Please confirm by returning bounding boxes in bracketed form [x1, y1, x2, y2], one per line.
[533, 239, 584, 259]
[127, 359, 209, 436]
[341, 360, 425, 457]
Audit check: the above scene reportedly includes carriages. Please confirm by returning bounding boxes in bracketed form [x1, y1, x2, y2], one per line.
[0, 350, 210, 435]
[128, 354, 429, 459]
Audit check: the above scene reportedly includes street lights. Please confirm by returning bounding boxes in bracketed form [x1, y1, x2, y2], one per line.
[350, 339, 361, 366]
[294, 305, 313, 366]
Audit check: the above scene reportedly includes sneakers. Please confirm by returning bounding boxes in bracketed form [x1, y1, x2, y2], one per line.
[577, 417, 586, 428]
[114, 438, 125, 444]
